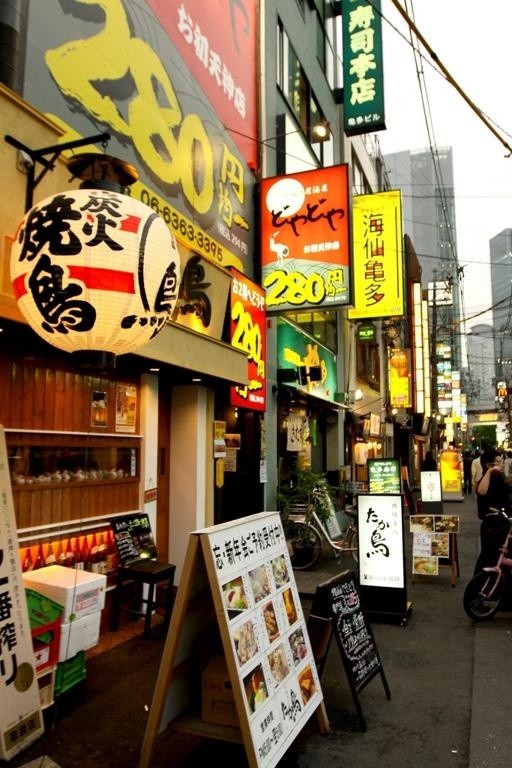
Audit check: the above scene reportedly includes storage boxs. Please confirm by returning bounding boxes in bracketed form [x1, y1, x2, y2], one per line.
[20, 563, 107, 698]
[199, 654, 242, 728]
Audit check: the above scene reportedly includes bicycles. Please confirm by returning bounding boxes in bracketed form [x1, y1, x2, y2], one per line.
[281, 487, 359, 571]
[462, 507, 511, 623]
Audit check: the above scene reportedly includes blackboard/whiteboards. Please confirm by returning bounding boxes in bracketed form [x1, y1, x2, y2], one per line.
[325, 571, 381, 692]
[415, 526, 454, 568]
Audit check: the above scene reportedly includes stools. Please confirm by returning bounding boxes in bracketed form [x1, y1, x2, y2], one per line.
[109, 559, 176, 642]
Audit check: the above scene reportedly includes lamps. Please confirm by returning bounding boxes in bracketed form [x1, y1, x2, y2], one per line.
[271, 365, 320, 395]
[334, 387, 364, 407]
[353, 439, 370, 468]
[387, 408, 399, 418]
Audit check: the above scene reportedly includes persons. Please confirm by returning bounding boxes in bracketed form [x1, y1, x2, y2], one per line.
[462, 439, 512, 574]
[419, 450, 437, 471]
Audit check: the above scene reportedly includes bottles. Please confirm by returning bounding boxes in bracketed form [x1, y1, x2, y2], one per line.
[18, 531, 119, 575]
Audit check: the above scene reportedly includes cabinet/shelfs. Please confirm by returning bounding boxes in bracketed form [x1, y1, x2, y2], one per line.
[3, 430, 144, 594]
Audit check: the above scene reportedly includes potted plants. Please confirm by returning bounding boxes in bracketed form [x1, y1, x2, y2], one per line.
[274, 466, 331, 546]
